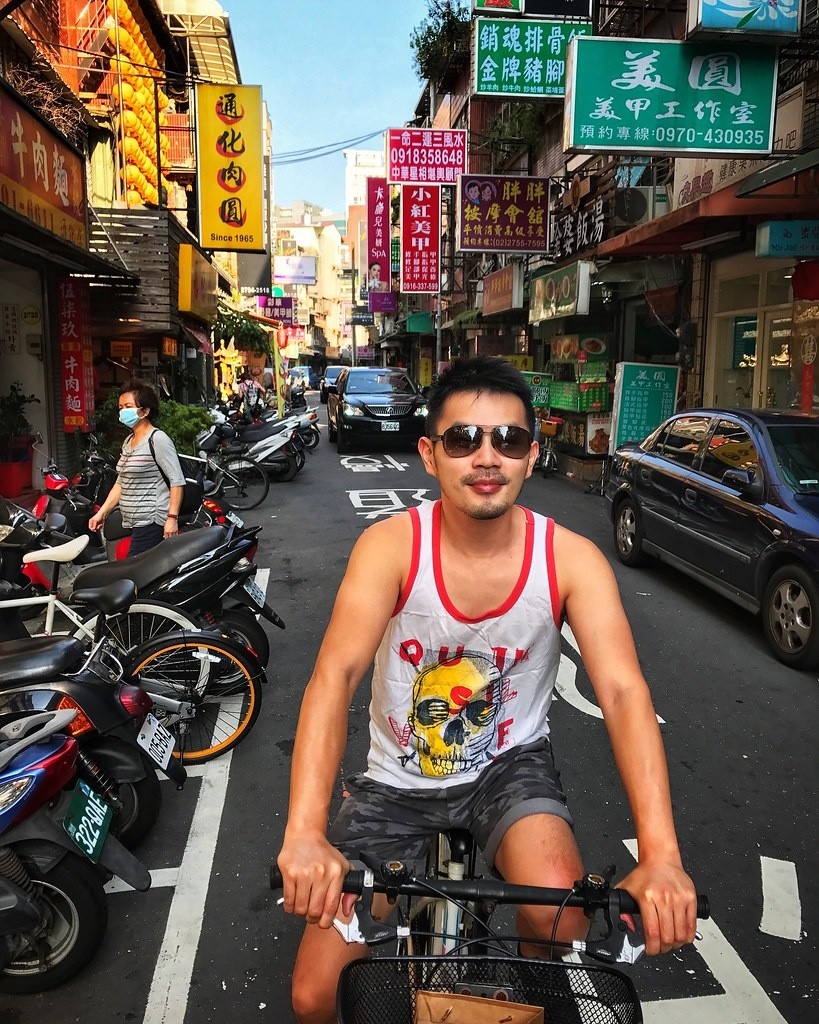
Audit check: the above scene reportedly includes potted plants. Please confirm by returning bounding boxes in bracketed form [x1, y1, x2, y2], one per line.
[0, 380, 42, 500]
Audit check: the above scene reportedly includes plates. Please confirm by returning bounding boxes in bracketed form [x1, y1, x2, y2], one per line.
[580, 338, 606, 355]
[546, 278, 555, 301]
[562, 275, 570, 298]
[557, 341, 563, 358]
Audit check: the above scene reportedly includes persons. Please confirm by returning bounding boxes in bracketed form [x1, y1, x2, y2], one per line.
[88, 382, 187, 557]
[368, 261, 389, 292]
[238, 373, 266, 420]
[275, 356, 698, 1024]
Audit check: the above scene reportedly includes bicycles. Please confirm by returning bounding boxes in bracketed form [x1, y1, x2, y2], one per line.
[269, 832, 711, 1024]
[538, 418, 559, 477]
[0, 535, 268, 789]
[178, 445, 271, 510]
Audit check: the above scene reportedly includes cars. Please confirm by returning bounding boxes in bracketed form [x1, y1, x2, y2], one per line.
[327, 367, 430, 454]
[604, 407, 819, 673]
[319, 366, 353, 402]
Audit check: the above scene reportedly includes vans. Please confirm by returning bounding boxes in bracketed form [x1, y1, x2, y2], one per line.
[292, 366, 317, 387]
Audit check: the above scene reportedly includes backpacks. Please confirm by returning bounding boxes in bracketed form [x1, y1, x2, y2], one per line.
[126, 429, 204, 515]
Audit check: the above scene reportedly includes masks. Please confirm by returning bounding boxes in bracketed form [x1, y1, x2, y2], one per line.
[119, 407, 144, 428]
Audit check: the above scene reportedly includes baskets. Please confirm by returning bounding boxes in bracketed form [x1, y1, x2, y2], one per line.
[333, 954, 645, 1024]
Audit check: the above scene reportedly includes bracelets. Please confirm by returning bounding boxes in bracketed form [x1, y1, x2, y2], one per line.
[167, 514, 179, 519]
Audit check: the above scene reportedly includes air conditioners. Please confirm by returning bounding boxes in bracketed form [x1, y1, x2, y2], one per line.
[614, 185, 671, 227]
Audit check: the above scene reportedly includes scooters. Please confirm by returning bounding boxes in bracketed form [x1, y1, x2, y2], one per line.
[196, 388, 322, 483]
[0, 492, 286, 698]
[0, 634, 188, 993]
[33, 425, 247, 545]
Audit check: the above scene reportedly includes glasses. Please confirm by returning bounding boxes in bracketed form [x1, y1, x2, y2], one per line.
[430, 425, 532, 459]
